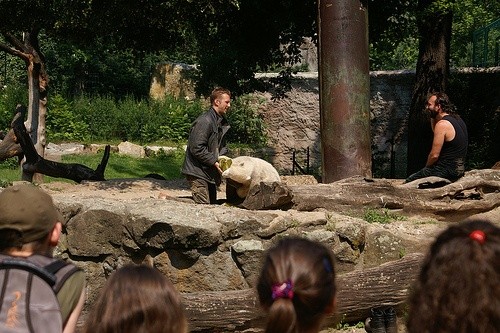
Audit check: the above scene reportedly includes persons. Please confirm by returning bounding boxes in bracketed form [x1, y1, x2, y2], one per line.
[403, 220, 500, 333]
[405, 92, 468, 183]
[257, 237, 337, 333]
[0, 184, 86, 333]
[181, 87, 231, 204]
[83, 262, 188, 333]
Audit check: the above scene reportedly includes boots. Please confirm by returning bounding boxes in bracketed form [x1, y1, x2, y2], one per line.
[383, 306, 398, 333]
[365, 307, 386, 333]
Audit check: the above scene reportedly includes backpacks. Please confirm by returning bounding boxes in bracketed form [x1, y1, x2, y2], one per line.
[0, 251, 83, 333]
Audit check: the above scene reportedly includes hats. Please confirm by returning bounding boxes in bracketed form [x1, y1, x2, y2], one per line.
[0, 183, 67, 245]
